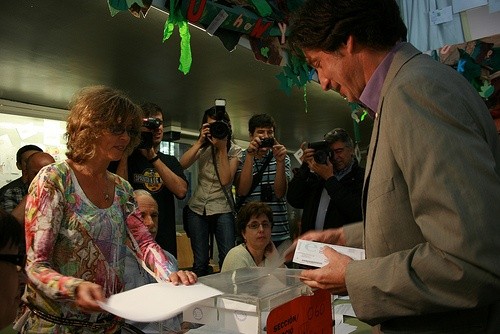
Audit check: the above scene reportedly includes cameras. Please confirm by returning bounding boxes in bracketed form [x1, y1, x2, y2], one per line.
[204, 99, 231, 145]
[258, 138, 274, 147]
[308, 140, 331, 165]
[138, 118, 162, 150]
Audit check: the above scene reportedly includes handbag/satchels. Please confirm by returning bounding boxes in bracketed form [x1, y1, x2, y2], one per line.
[233, 212, 245, 233]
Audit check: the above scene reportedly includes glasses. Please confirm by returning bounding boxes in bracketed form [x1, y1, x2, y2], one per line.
[0, 250, 27, 273]
[246, 220, 272, 231]
[93, 122, 140, 137]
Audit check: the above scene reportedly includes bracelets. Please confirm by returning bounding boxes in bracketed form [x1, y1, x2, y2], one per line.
[149, 154, 159, 163]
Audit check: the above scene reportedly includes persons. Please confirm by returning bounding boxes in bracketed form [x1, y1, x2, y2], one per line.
[233, 114, 291, 249]
[0, 152, 55, 308]
[120, 189, 196, 334]
[10, 86, 198, 334]
[107, 102, 188, 261]
[179, 105, 243, 277]
[221, 202, 282, 273]
[283, 0, 500, 334]
[0, 145, 43, 197]
[286, 128, 365, 236]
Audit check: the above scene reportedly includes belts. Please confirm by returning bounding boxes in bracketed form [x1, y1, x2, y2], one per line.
[25, 302, 146, 334]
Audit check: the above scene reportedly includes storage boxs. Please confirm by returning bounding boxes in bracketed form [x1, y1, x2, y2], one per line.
[183, 266, 334, 334]
